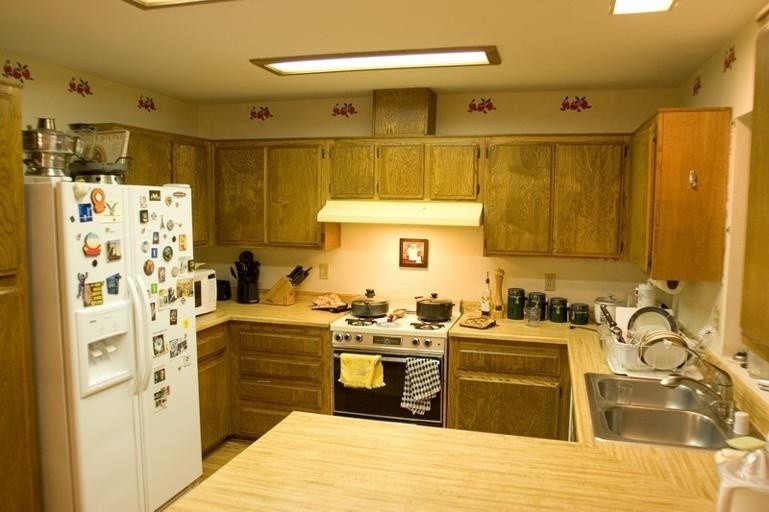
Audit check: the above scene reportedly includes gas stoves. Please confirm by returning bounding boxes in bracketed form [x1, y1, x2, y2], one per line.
[329, 309, 461, 355]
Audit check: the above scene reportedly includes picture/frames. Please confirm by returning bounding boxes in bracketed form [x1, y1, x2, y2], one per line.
[399, 238, 428, 267]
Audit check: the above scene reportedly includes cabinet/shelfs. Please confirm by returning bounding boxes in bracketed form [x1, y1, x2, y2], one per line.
[624, 106, 732, 281]
[197, 323, 232, 456]
[69, 122, 217, 248]
[446, 337, 569, 440]
[0, 77, 44, 512]
[327, 136, 483, 202]
[484, 134, 628, 260]
[227, 320, 332, 440]
[217, 136, 340, 252]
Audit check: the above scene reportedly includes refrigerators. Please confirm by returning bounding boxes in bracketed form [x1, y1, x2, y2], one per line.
[21, 182, 206, 510]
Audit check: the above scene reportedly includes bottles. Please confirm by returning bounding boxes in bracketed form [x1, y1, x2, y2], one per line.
[633, 282, 658, 306]
[507, 288, 525, 320]
[570, 303, 589, 325]
[481, 271, 493, 317]
[528, 291, 546, 321]
[550, 297, 568, 323]
[717, 448, 769, 511]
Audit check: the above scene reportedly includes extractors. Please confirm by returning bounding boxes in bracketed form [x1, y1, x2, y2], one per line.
[316, 199, 486, 229]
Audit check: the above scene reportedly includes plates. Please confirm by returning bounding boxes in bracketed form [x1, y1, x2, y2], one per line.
[757, 380, 769, 391]
[627, 305, 677, 333]
[640, 330, 689, 371]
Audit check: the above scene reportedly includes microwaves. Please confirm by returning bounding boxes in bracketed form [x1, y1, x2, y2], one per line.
[193, 269, 218, 316]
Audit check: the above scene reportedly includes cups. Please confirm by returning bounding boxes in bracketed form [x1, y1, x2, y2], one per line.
[524, 308, 541, 325]
[732, 411, 752, 436]
[746, 350, 769, 379]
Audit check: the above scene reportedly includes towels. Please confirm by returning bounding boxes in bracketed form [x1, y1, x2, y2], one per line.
[400, 357, 441, 415]
[338, 353, 386, 390]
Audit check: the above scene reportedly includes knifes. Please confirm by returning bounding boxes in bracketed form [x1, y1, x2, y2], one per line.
[287, 265, 314, 286]
[600, 303, 626, 344]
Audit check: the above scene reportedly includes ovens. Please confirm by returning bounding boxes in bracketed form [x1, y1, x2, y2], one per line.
[332, 350, 445, 428]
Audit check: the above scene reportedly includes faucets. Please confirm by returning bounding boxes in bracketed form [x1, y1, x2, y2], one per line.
[660, 358, 735, 427]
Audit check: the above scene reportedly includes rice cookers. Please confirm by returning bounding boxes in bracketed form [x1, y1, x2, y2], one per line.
[592, 293, 626, 326]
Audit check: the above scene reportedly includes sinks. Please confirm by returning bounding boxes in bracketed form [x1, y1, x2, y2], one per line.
[589, 405, 767, 449]
[584, 373, 721, 410]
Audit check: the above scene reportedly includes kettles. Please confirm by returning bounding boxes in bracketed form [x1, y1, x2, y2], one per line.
[228, 250, 261, 305]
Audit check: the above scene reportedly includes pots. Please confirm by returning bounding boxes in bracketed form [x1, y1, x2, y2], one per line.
[21, 118, 81, 182]
[351, 288, 389, 317]
[65, 153, 132, 184]
[413, 292, 455, 320]
